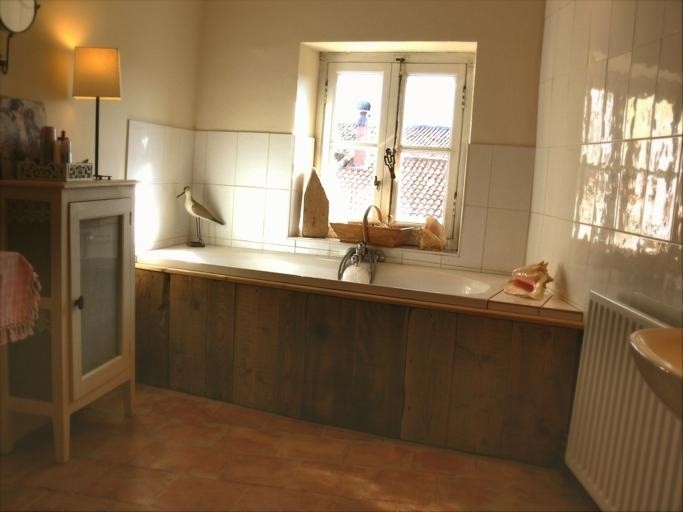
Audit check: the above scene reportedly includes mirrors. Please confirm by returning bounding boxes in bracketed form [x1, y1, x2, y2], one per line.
[1, 0, 36, 34]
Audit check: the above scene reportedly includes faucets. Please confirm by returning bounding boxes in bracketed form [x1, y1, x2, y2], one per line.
[349, 204, 384, 266]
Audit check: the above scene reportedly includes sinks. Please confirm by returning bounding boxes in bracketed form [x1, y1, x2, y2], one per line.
[627, 327, 682, 420]
[330, 222, 415, 246]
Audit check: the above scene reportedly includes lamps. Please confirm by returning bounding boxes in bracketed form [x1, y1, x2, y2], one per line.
[73, 48, 121, 180]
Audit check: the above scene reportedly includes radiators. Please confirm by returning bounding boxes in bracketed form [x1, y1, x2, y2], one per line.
[561, 288, 682, 512]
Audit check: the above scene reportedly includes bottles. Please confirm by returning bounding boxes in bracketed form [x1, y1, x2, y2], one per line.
[53, 130, 72, 167]
[38, 125, 57, 166]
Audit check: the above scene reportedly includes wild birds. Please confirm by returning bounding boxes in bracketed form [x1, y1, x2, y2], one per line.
[176, 185, 224, 244]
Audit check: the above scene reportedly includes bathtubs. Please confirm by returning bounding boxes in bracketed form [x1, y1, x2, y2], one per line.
[135, 241, 514, 310]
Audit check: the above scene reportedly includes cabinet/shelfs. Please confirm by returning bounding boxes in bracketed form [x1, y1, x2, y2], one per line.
[2, 188, 136, 468]
